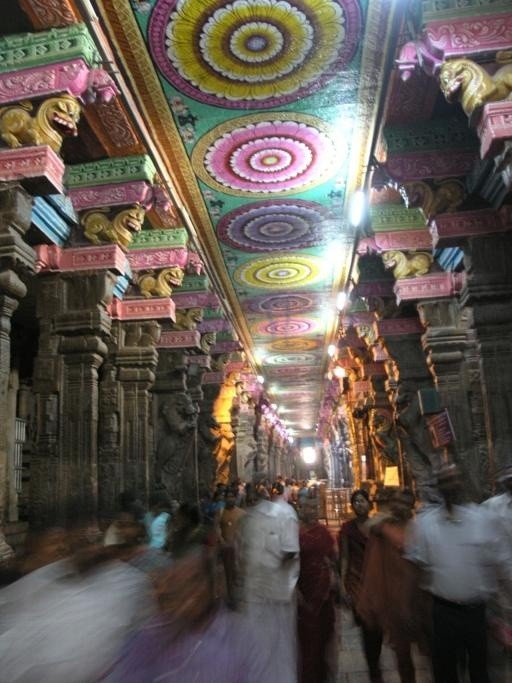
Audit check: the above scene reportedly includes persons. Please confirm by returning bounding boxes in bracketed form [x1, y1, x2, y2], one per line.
[0, 463, 512, 683]
